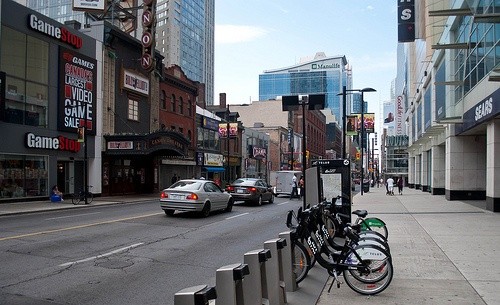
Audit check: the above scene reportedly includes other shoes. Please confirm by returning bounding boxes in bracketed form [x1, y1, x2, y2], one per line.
[61, 198, 64, 201]
[389, 193, 393, 195]
[398, 192, 402, 194]
[290, 198, 291, 200]
[298, 198, 300, 200]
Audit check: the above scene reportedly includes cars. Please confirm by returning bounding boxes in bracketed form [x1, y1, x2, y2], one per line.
[160, 179, 233, 218]
[226, 178, 274, 207]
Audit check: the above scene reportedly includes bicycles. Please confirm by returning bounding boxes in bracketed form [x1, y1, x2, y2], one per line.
[286, 193, 393, 295]
[72, 185, 93, 205]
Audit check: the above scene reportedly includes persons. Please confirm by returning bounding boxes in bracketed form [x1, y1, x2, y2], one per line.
[52, 184, 65, 201]
[384, 112, 394, 122]
[290, 172, 299, 200]
[299, 175, 305, 197]
[380, 174, 404, 195]
[171, 172, 221, 186]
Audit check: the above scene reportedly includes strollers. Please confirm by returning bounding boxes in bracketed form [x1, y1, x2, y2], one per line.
[386, 184, 394, 196]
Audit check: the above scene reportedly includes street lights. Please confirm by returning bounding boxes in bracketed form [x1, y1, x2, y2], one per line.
[343, 85, 376, 158]
[63, 101, 88, 204]
